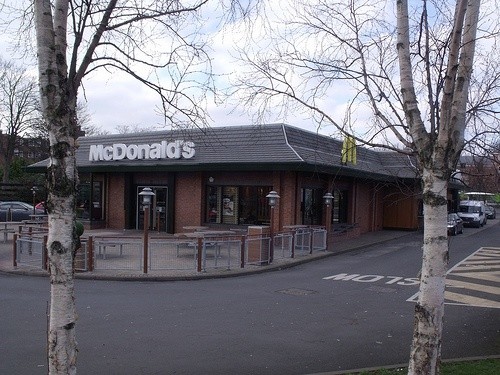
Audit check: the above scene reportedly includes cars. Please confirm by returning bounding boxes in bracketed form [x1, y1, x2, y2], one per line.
[446, 212, 464, 236]
[484, 206, 497, 219]
[0, 201, 49, 222]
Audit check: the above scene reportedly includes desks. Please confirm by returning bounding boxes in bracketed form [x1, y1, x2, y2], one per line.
[0, 221, 25, 243]
[174, 225, 247, 260]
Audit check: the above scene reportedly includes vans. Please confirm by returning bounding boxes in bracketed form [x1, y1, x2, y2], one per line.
[455, 200, 487, 228]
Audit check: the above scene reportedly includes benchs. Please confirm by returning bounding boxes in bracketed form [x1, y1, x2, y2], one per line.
[0, 228, 16, 233]
[95, 240, 130, 260]
[186, 243, 224, 259]
